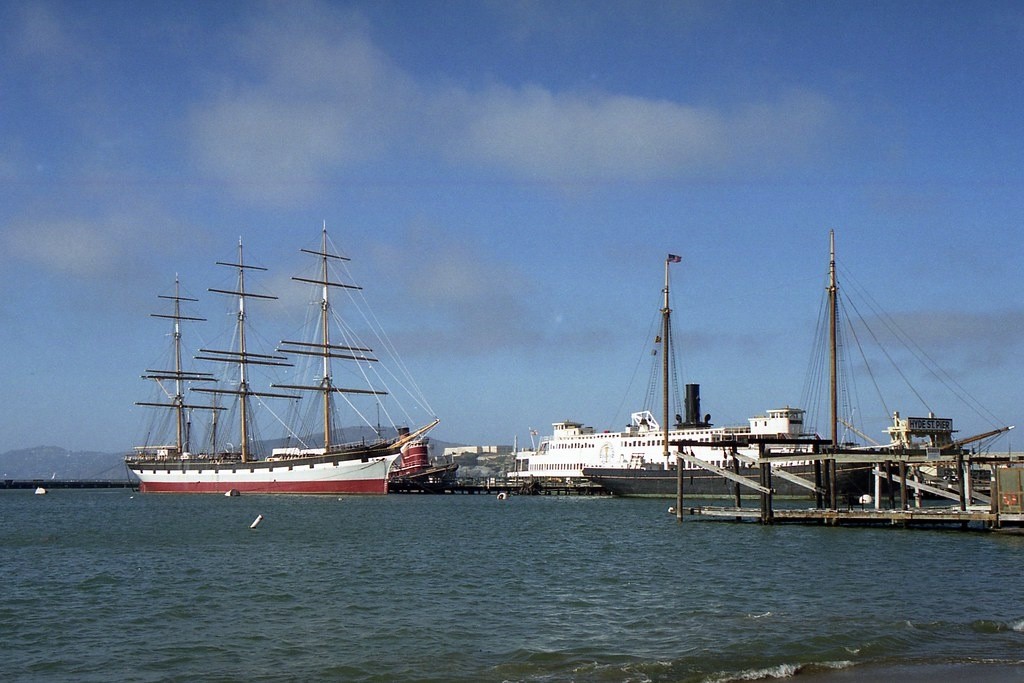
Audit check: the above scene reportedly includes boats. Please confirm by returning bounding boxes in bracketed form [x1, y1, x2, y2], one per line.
[499, 381, 893, 485]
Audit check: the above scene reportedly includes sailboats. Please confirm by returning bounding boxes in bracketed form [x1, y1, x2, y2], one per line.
[579, 227, 1016, 501]
[122, 219, 442, 496]
